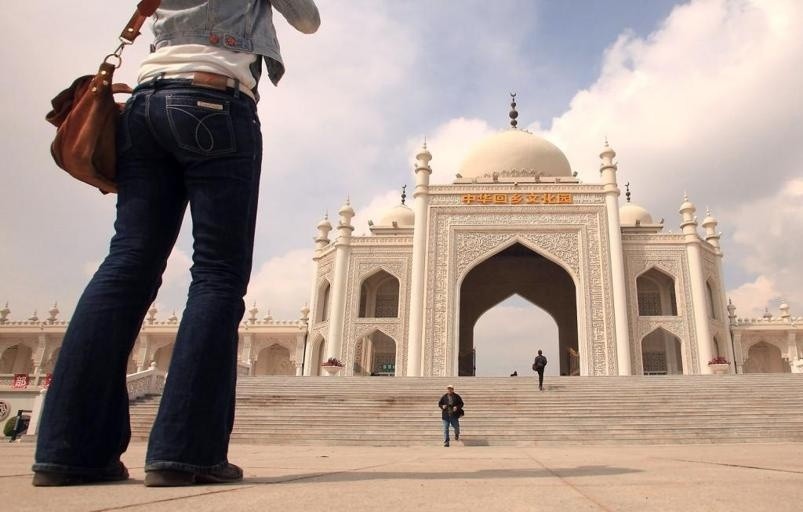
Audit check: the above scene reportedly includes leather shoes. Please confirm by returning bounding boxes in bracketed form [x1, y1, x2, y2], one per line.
[33, 466, 128, 485]
[143, 459, 243, 486]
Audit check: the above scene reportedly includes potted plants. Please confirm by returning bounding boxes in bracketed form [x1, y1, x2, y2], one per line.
[706, 356, 731, 378]
[321, 357, 344, 375]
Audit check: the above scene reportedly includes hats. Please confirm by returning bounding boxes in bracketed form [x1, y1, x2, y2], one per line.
[447, 385, 454, 389]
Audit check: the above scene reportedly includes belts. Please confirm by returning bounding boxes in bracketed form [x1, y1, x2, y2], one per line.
[139, 72, 256, 101]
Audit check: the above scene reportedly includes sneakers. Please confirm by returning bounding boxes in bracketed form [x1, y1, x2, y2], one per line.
[455, 434, 459, 440]
[444, 441, 448, 447]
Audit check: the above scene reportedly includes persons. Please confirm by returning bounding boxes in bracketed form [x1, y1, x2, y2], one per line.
[533, 348, 547, 392]
[30, 2, 320, 485]
[438, 384, 466, 448]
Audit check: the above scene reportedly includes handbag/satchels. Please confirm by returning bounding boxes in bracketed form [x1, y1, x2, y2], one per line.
[46, 0, 162, 194]
[456, 409, 464, 417]
